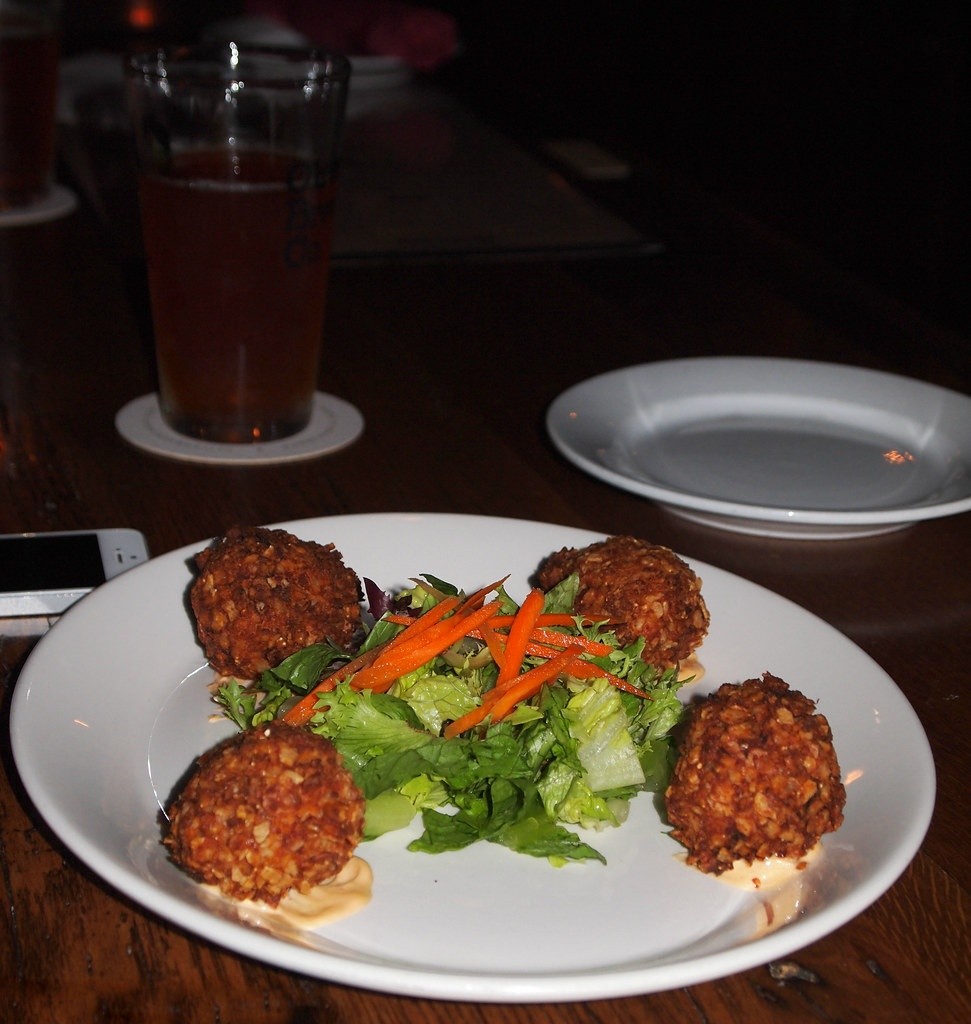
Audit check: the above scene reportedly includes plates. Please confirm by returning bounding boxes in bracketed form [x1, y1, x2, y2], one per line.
[545, 357, 971, 540]
[227, 58, 419, 117]
[64, 51, 295, 91]
[9, 512, 936, 1003]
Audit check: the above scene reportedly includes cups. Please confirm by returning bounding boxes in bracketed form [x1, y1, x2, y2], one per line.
[0, 5, 63, 209]
[124, 41, 352, 445]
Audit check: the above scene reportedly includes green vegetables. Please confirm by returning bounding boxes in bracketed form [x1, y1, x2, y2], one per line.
[210, 572, 694, 864]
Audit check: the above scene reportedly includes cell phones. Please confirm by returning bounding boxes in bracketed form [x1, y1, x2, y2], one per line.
[0, 527, 147, 620]
